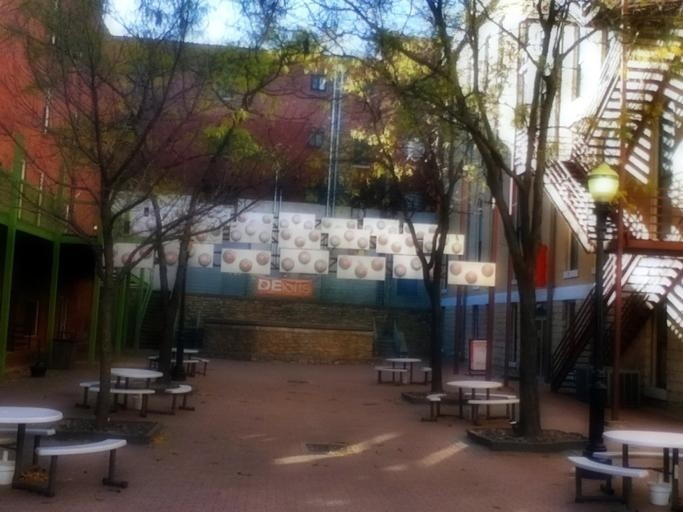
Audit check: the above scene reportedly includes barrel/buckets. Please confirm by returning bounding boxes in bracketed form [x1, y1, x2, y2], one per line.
[131, 394, 142, 410]
[0, 449, 16, 485]
[648, 471, 672, 506]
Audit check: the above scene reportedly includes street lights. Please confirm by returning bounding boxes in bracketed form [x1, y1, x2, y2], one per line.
[579, 160, 620, 479]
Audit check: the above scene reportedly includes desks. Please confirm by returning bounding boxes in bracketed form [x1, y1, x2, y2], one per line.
[387, 357, 422, 384]
[171, 348, 198, 373]
[0, 406, 64, 489]
[602, 430, 683, 512]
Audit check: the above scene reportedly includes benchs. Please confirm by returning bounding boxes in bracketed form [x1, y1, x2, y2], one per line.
[76, 356, 209, 416]
[567, 457, 648, 512]
[35, 438, 127, 497]
[376, 365, 520, 423]
[593, 451, 683, 494]
[0, 428, 56, 466]
